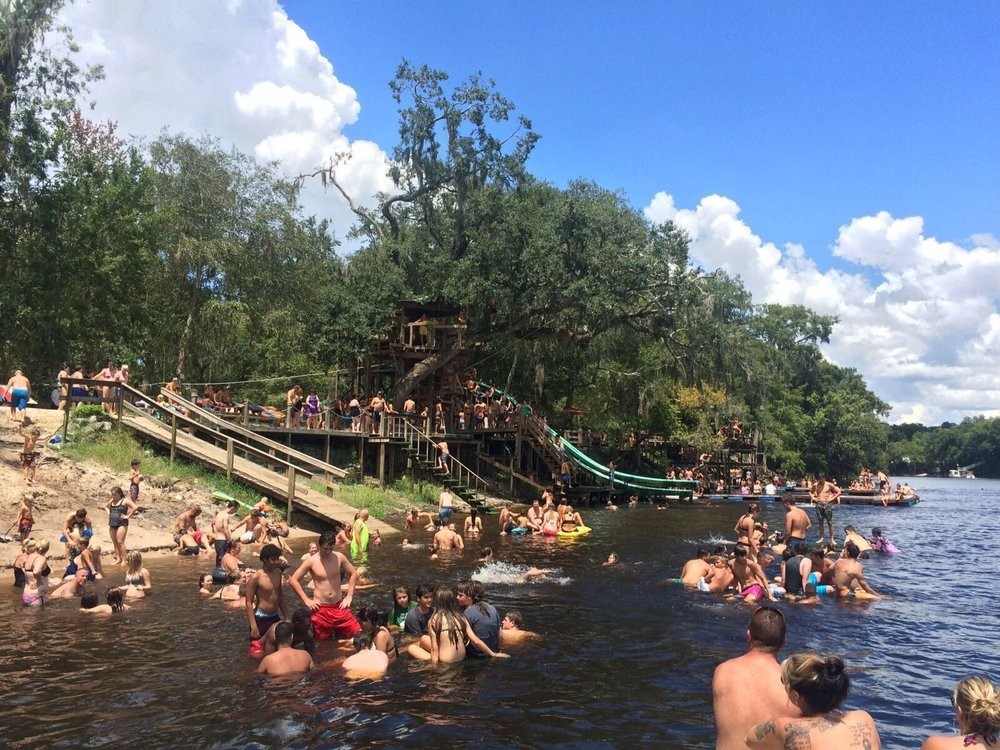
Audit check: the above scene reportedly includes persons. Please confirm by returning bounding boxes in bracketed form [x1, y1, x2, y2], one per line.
[711, 607, 802, 750]
[921, 676, 1000, 750]
[128, 459, 144, 515]
[2, 370, 32, 426]
[408, 290, 499, 351]
[13, 487, 51, 613]
[596, 416, 920, 608]
[743, 652, 882, 750]
[172, 485, 585, 681]
[51, 481, 154, 617]
[16, 423, 41, 484]
[43, 357, 604, 497]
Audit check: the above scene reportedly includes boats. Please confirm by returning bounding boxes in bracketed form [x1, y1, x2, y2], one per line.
[947, 463, 976, 480]
